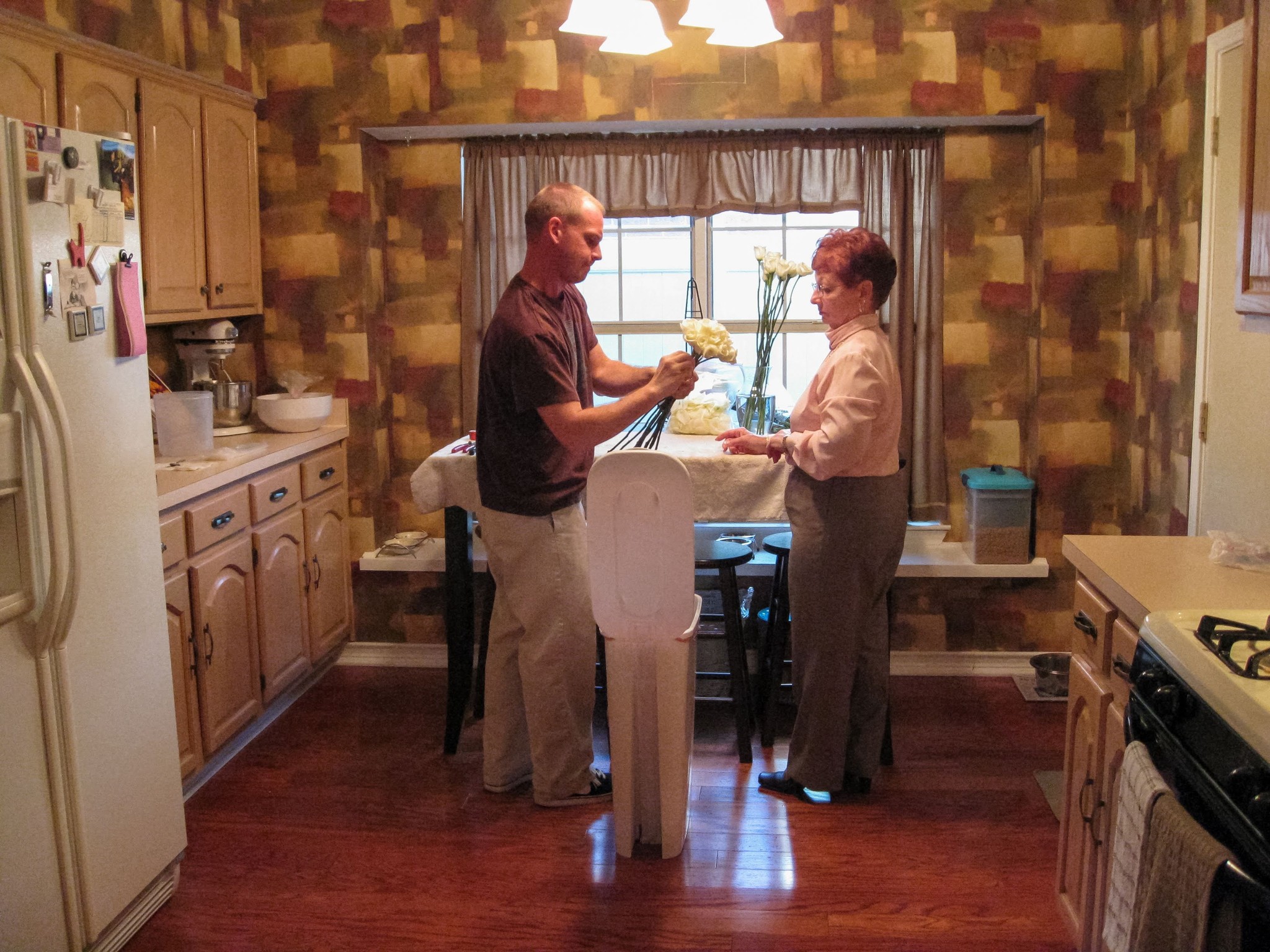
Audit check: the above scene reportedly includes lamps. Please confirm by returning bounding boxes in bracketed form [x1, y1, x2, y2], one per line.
[559, 0, 785, 57]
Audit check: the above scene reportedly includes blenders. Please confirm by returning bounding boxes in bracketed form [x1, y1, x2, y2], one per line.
[170, 317, 260, 436]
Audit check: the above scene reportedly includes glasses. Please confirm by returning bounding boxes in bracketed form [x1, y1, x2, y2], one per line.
[812, 282, 848, 298]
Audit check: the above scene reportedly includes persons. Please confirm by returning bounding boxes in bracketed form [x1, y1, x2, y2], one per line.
[715, 227, 908, 799]
[100, 150, 126, 190]
[476, 182, 699, 807]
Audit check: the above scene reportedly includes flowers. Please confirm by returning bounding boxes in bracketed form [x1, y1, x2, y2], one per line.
[740, 245, 812, 438]
[608, 316, 738, 449]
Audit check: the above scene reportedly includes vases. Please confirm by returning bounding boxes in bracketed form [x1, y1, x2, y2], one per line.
[736, 364, 775, 436]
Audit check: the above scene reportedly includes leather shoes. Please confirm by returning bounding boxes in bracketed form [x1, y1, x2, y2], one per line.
[758, 770, 805, 795]
[841, 773, 873, 792]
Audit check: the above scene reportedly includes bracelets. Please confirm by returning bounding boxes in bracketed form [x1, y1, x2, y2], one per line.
[783, 433, 791, 452]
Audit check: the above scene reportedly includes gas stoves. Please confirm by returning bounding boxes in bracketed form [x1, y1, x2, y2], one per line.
[1130, 607, 1270, 847]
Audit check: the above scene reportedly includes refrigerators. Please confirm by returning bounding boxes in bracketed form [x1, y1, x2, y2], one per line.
[1, 117, 188, 951]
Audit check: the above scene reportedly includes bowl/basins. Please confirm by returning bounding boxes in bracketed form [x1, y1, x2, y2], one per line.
[1030, 652, 1070, 697]
[192, 375, 253, 431]
[385, 531, 428, 555]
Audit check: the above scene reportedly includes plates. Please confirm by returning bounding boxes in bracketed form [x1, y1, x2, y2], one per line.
[257, 391, 336, 433]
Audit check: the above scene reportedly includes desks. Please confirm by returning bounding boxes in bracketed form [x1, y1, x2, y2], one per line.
[358, 538, 1050, 714]
[408, 431, 794, 763]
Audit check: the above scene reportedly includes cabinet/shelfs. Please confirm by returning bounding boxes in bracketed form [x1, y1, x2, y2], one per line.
[0, 7, 265, 327]
[157, 437, 356, 805]
[1052, 570, 1141, 952]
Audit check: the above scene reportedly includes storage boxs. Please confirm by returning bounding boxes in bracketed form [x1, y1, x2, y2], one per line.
[401, 581, 448, 644]
[961, 465, 1037, 566]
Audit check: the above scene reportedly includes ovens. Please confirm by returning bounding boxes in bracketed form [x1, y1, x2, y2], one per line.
[1123, 688, 1270, 951]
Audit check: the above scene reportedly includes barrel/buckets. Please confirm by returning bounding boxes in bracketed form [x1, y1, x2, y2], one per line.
[153, 389, 214, 460]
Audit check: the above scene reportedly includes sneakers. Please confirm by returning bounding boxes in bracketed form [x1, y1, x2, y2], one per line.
[484, 771, 533, 793]
[534, 768, 612, 807]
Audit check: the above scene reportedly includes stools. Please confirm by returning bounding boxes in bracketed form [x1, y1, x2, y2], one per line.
[687, 532, 898, 768]
